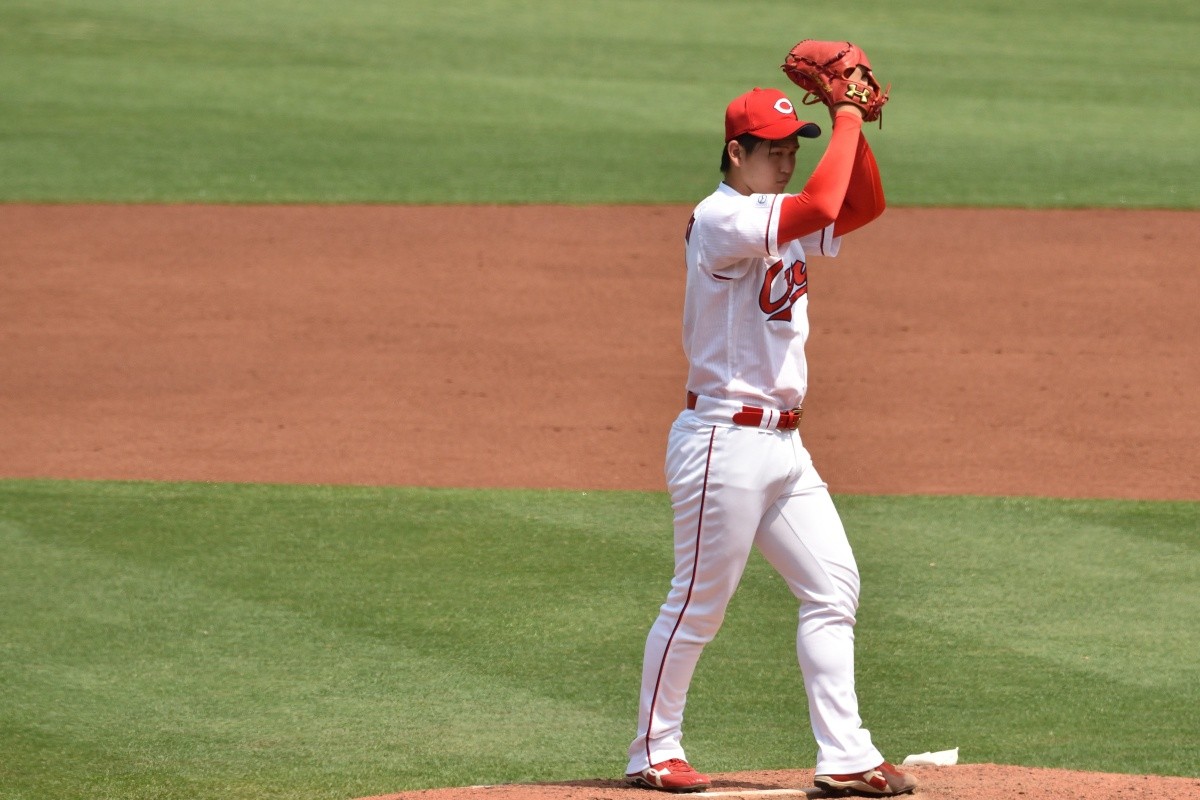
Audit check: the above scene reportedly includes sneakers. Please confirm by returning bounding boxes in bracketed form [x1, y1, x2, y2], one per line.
[814, 761, 918, 796]
[624, 758, 711, 793]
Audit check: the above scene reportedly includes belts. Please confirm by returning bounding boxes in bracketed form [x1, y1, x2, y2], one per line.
[687, 391, 804, 430]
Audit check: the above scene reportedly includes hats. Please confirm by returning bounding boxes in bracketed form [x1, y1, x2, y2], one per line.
[724, 88, 822, 143]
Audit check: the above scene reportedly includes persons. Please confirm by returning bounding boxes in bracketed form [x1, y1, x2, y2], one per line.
[624, 39, 918, 795]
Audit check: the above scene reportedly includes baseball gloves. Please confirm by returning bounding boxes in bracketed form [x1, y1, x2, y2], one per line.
[781, 39, 885, 122]
